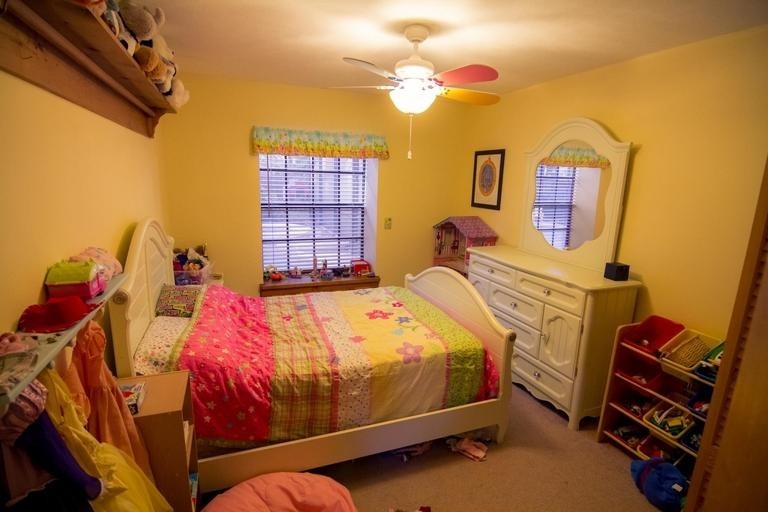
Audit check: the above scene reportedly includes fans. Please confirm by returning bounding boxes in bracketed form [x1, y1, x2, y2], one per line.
[324, 26, 501, 105]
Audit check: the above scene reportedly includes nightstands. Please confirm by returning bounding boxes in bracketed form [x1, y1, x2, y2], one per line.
[115, 369, 200, 512]
[206, 271, 224, 286]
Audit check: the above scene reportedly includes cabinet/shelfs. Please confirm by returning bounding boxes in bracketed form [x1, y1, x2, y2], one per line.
[464, 244, 642, 431]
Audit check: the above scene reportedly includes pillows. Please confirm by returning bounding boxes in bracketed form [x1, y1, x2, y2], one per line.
[136, 316, 190, 375]
[155, 283, 200, 316]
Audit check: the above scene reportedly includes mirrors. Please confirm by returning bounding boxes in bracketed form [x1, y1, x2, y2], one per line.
[516, 115, 632, 272]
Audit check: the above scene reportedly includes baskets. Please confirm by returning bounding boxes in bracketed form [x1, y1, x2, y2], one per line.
[657, 327, 723, 383]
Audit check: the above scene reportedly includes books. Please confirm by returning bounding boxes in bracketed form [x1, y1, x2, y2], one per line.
[119, 381, 146, 414]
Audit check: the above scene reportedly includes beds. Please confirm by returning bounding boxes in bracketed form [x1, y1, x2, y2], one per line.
[108, 218, 516, 497]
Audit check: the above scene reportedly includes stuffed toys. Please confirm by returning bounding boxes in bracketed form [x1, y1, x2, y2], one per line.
[76, 0, 191, 108]
[629, 456, 689, 512]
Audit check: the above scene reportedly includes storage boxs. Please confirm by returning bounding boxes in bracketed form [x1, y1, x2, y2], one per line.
[606, 315, 724, 473]
[174, 257, 210, 285]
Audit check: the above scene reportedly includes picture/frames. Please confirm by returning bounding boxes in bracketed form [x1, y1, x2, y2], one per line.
[470, 149, 505, 210]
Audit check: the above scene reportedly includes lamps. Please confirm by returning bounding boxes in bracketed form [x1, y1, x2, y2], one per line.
[387, 89, 438, 160]
